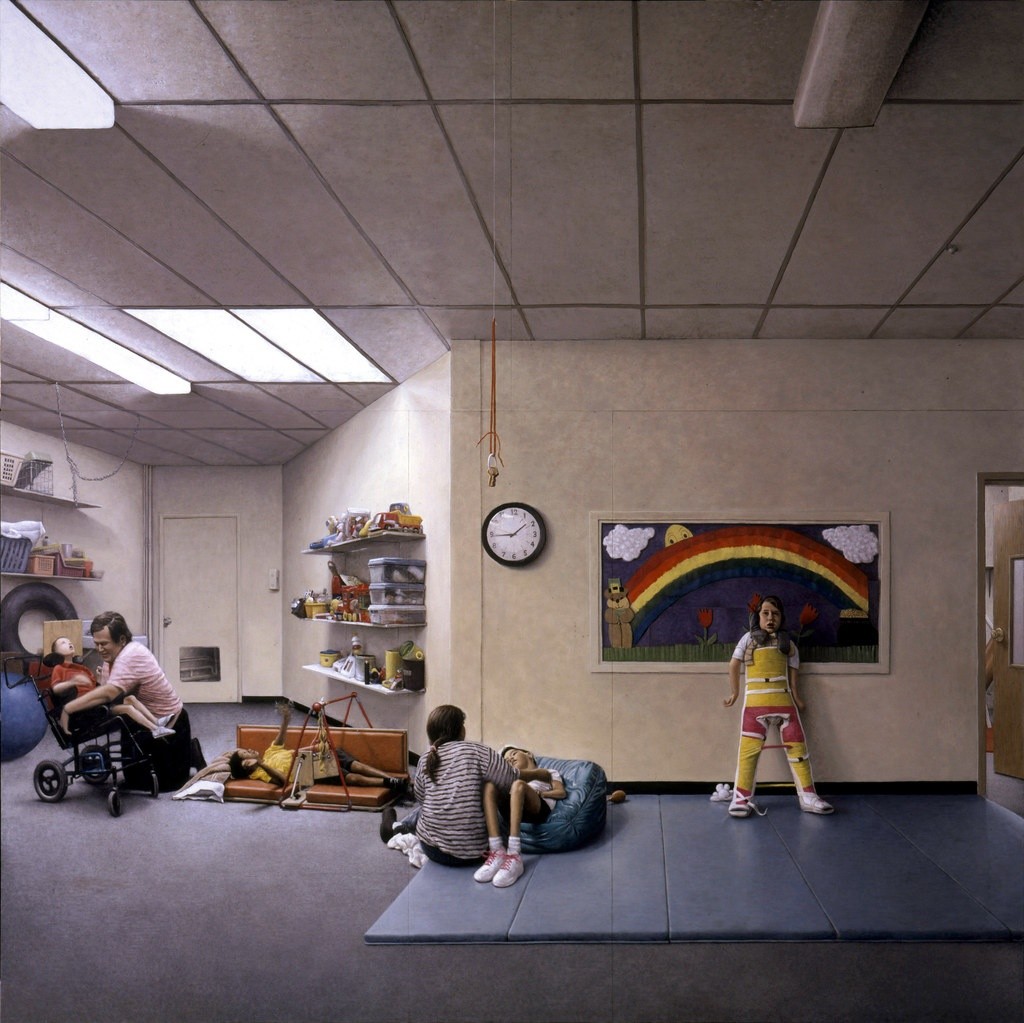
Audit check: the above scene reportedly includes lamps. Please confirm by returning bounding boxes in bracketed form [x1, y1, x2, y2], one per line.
[0, 280, 192, 395]
[0, 0, 116, 131]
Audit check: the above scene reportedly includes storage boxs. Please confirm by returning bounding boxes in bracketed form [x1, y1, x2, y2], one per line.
[0, 450, 25, 487]
[367, 557, 426, 624]
[0, 537, 33, 573]
[25, 543, 59, 576]
[304, 603, 329, 618]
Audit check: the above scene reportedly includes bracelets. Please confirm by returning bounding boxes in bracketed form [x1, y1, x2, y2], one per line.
[62, 705, 71, 716]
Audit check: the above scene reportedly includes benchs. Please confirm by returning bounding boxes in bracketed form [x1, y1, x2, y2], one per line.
[223, 724, 408, 813]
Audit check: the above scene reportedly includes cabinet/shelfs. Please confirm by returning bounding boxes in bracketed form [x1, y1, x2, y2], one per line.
[0, 483, 102, 582]
[300, 531, 427, 696]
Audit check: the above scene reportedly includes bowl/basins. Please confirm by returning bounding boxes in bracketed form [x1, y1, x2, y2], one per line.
[320, 651, 341, 666]
[91, 569, 104, 578]
[305, 603, 329, 617]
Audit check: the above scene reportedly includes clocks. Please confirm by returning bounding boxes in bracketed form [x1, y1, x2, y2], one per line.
[480, 502, 546, 567]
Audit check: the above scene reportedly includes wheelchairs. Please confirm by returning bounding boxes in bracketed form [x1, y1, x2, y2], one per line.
[4, 652, 169, 817]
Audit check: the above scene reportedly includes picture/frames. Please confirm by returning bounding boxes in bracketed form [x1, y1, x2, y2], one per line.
[587, 509, 892, 675]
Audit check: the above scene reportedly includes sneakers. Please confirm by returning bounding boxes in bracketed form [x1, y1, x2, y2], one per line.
[380, 806, 397, 842]
[472, 851, 524, 888]
[152, 713, 176, 739]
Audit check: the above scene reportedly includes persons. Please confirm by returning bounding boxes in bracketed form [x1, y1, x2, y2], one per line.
[391, 704, 552, 869]
[59, 611, 207, 792]
[50, 635, 176, 739]
[474, 745, 566, 889]
[721, 594, 834, 819]
[225, 706, 411, 791]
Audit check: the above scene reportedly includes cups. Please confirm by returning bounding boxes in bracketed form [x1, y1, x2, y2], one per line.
[62, 544, 72, 560]
[83, 562, 93, 577]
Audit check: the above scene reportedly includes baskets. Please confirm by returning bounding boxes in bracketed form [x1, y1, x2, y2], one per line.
[0, 537, 87, 577]
[0, 450, 24, 486]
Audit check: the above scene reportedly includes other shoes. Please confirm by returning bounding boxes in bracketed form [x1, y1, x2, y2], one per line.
[728, 801, 754, 817]
[384, 775, 411, 796]
[799, 795, 835, 815]
[190, 738, 207, 770]
